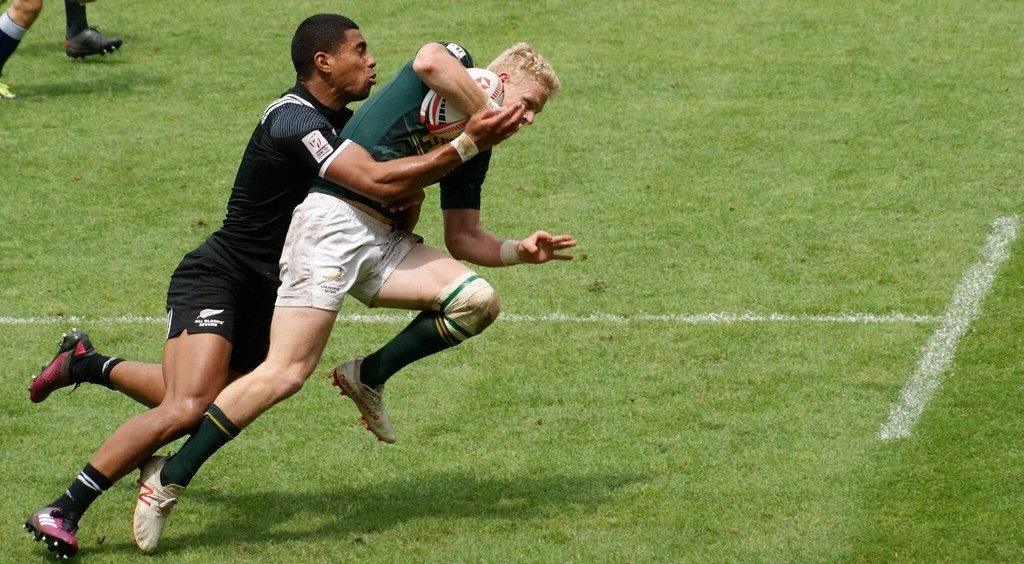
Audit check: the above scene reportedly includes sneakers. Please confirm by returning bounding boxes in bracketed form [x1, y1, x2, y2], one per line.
[65, 28, 122, 61]
[24, 503, 81, 560]
[133, 455, 186, 555]
[332, 360, 396, 446]
[30, 326, 95, 406]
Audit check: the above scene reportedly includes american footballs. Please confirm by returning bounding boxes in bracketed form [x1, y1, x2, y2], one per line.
[419, 68, 505, 139]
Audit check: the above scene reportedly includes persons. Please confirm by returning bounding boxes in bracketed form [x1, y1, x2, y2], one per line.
[23, 13, 576, 559]
[64, 0, 123, 59]
[0, 0, 42, 100]
